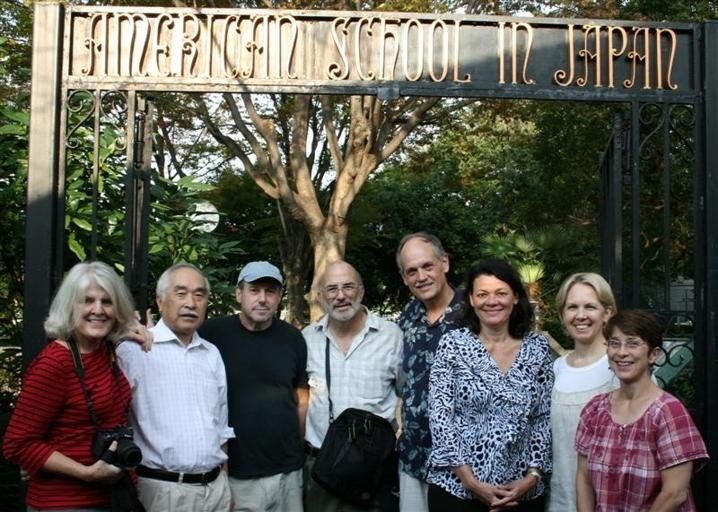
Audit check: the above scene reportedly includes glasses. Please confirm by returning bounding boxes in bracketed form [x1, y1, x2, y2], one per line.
[604, 340, 657, 349]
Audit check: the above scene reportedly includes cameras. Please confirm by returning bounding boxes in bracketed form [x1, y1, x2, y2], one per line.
[89, 424, 144, 471]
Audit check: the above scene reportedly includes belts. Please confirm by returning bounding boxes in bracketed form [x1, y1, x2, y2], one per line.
[135, 466, 224, 484]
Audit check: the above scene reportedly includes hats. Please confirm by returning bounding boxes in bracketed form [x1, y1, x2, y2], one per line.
[236, 260, 284, 288]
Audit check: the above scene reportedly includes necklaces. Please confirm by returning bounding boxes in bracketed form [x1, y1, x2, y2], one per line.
[478, 332, 522, 369]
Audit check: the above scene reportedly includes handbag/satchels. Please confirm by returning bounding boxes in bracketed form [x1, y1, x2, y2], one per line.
[310, 337, 397, 507]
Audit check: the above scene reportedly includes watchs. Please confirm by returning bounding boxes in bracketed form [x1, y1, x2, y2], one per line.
[525, 471, 541, 487]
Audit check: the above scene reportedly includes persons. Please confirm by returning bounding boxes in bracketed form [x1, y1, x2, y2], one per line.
[546, 271, 617, 512]
[2, 260, 153, 511]
[422, 258, 555, 512]
[293, 259, 405, 512]
[574, 307, 710, 512]
[397, 231, 467, 512]
[20, 263, 237, 512]
[195, 261, 308, 512]
